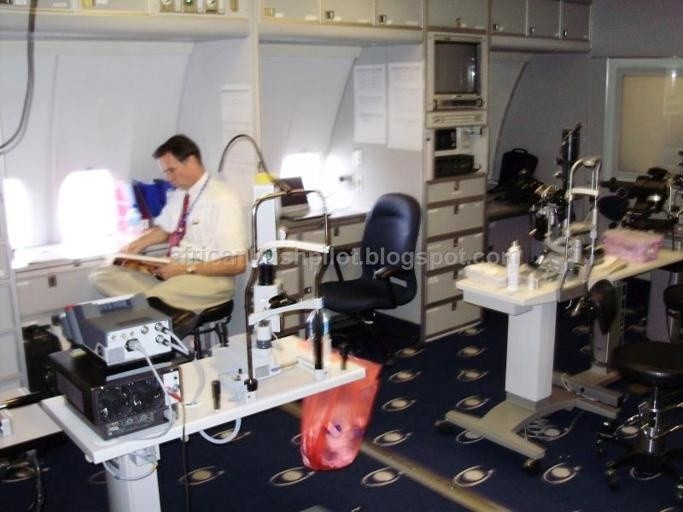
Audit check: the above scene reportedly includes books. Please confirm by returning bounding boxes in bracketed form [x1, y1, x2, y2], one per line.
[106, 253, 171, 278]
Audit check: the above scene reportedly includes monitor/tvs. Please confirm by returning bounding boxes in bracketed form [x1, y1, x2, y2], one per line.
[432, 35, 479, 109]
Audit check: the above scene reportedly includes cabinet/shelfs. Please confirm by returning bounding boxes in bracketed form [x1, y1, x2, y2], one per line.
[353, 31, 489, 345]
[15, 240, 170, 351]
[487, 189, 540, 263]
[273, 209, 367, 339]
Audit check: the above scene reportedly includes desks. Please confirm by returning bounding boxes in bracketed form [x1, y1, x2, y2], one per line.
[433, 249, 683, 478]
[38, 333, 366, 512]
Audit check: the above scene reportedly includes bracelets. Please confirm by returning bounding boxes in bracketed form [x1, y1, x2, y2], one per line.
[186, 266, 196, 274]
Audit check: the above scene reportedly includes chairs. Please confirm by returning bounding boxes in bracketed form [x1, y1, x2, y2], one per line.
[584, 279, 683, 504]
[170, 298, 234, 360]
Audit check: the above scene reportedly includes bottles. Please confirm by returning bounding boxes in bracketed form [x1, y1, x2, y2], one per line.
[572, 239, 581, 264]
[255, 319, 271, 349]
[306, 307, 329, 341]
[505, 239, 521, 293]
[0, 402, 13, 437]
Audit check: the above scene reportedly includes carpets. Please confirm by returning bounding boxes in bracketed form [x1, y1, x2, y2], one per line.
[0, 408, 476, 512]
[291, 306, 682, 512]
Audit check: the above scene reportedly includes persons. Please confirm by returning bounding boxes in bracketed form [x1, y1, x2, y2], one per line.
[89, 133, 250, 328]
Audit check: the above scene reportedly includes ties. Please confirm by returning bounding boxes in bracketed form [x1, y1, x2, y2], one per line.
[167, 192, 189, 256]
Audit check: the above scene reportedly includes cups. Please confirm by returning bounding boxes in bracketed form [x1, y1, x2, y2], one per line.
[528, 279, 539, 290]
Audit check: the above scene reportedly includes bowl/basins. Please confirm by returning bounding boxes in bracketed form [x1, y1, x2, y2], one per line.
[434, 154, 472, 177]
